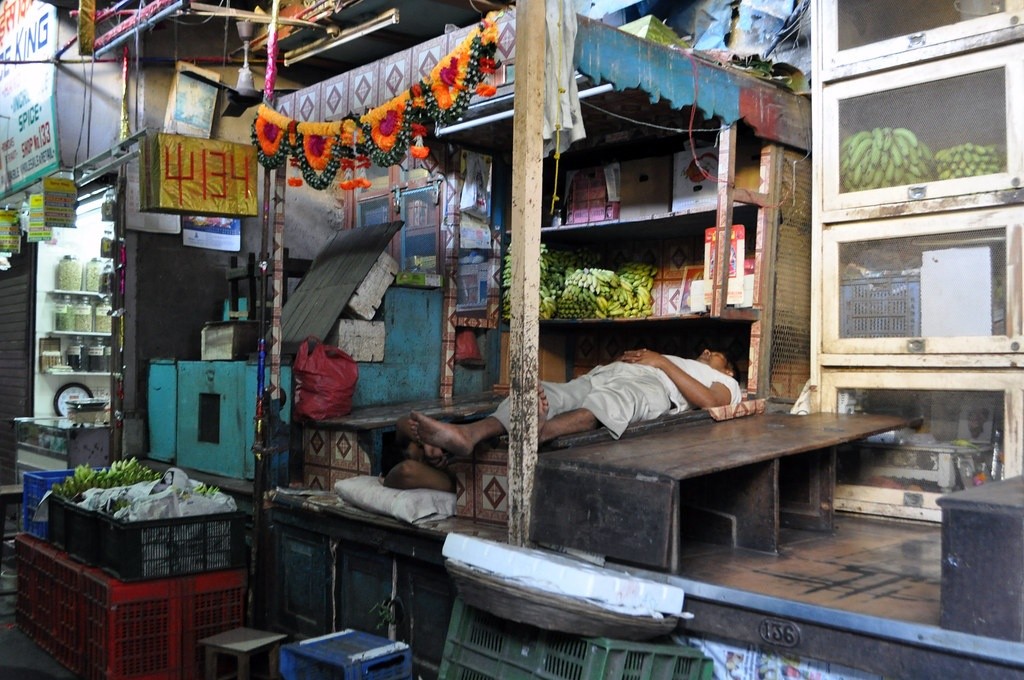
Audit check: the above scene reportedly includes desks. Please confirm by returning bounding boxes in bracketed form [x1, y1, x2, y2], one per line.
[10, 415, 112, 548]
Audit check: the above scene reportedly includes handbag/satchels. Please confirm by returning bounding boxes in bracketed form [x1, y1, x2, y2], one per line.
[292, 337, 359, 422]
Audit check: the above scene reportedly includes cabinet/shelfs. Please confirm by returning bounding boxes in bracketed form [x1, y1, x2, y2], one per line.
[47, 289, 115, 377]
[300, 380, 922, 573]
[807, 0, 1023, 526]
[935, 474, 1023, 642]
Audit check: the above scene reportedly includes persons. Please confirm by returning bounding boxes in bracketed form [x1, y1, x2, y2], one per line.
[379, 416, 457, 493]
[410, 348, 743, 457]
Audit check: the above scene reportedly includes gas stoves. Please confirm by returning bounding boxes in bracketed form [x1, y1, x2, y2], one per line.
[68, 409, 111, 428]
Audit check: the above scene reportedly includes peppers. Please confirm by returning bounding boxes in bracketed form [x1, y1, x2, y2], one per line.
[50, 456, 220, 513]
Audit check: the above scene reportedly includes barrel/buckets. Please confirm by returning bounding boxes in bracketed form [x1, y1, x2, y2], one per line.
[406, 202, 428, 228]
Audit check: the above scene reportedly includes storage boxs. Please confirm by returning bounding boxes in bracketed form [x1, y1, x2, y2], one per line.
[13, 466, 252, 680]
[438, 596, 715, 680]
[201, 320, 266, 361]
[565, 146, 757, 316]
[501, 332, 630, 390]
[329, 251, 445, 361]
[276, 629, 414, 680]
[145, 357, 293, 482]
[841, 274, 922, 340]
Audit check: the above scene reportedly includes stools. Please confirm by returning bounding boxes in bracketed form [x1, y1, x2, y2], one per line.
[198, 627, 287, 680]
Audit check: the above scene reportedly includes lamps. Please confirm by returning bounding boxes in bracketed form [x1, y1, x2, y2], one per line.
[284, 7, 400, 67]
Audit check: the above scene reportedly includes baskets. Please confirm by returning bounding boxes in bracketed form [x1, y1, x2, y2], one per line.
[49, 483, 244, 584]
[839, 275, 920, 338]
[439, 598, 704, 680]
[20, 466, 113, 541]
[15, 533, 249, 680]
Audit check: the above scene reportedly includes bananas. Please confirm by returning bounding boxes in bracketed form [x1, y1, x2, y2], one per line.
[839, 126, 1000, 193]
[502, 241, 657, 319]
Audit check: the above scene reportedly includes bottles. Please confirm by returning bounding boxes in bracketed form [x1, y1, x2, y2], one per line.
[102, 265, 114, 293]
[101, 230, 113, 258]
[102, 194, 115, 221]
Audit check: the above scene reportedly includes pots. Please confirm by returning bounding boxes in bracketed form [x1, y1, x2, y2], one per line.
[65, 398, 108, 410]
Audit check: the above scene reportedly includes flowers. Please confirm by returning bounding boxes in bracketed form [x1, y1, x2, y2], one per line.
[250, 14, 500, 191]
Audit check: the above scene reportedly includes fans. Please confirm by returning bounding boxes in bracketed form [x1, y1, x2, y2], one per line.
[179, 22, 302, 118]
